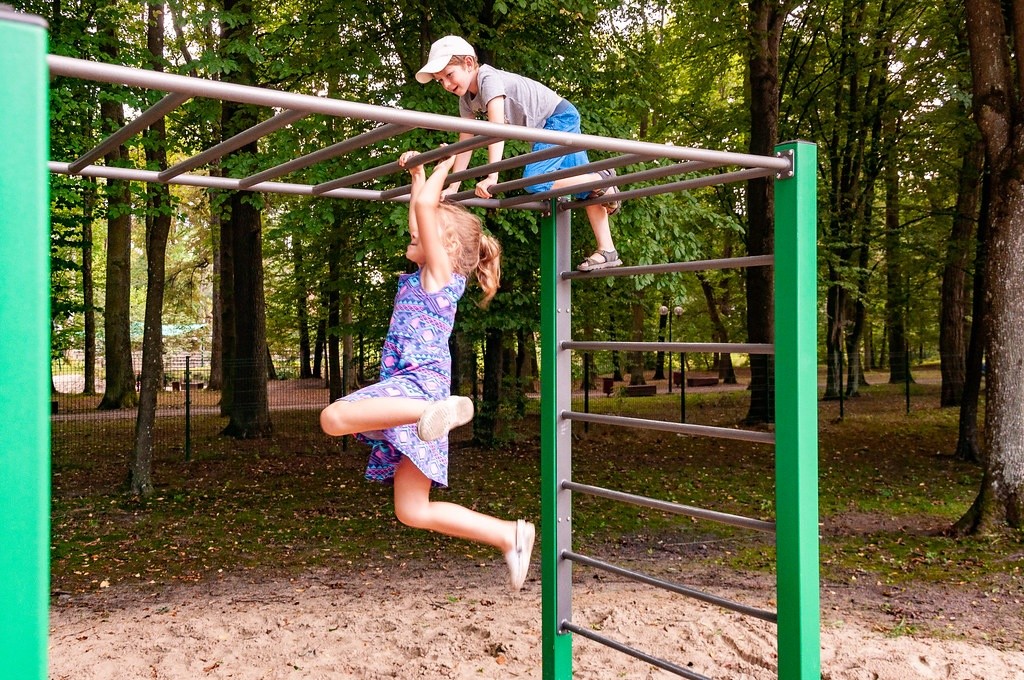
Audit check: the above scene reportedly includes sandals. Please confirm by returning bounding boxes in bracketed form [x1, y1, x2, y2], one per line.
[504, 519, 535, 590]
[591, 167, 622, 216]
[417, 395, 474, 441]
[577, 250, 623, 271]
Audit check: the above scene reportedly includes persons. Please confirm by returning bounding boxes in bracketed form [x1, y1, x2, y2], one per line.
[320, 142, 536, 591]
[414, 35, 623, 271]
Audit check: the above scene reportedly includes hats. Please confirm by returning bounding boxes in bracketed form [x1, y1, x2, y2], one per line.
[415, 36, 476, 85]
